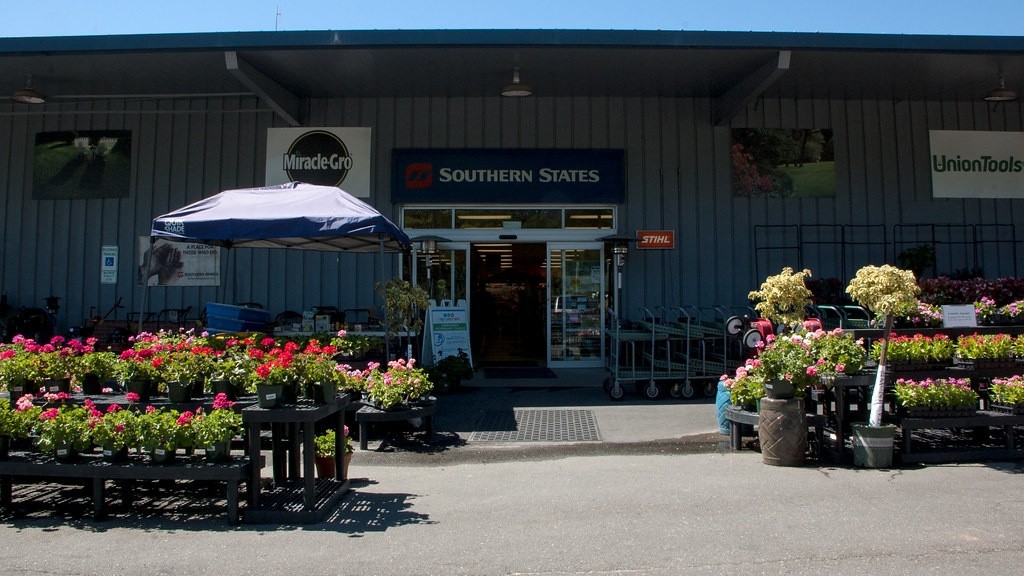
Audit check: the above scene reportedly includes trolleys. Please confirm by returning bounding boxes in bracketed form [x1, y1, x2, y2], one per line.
[603, 303, 882, 404]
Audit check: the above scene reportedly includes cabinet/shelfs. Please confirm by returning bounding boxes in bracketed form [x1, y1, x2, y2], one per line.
[725, 325, 1024, 462]
[0, 392, 437, 524]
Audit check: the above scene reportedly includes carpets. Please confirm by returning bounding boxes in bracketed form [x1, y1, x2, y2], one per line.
[483, 367, 557, 380]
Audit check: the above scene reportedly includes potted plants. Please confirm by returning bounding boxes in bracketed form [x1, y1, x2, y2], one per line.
[845, 264, 920, 469]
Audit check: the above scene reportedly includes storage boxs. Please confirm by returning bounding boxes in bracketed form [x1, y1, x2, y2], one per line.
[203, 301, 331, 336]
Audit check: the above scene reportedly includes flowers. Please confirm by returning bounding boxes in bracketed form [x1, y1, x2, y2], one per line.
[0, 386, 245, 450]
[718, 277, 1024, 409]
[0, 327, 354, 386]
[355, 358, 435, 408]
[425, 348, 479, 381]
[0, 393, 435, 451]
[313, 425, 355, 459]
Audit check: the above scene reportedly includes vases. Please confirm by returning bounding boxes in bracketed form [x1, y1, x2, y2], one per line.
[738, 399, 761, 415]
[887, 359, 942, 372]
[313, 452, 352, 479]
[441, 381, 461, 395]
[7, 372, 339, 410]
[954, 356, 1018, 370]
[373, 395, 408, 412]
[991, 400, 1024, 415]
[760, 377, 796, 399]
[0, 432, 231, 464]
[902, 405, 976, 417]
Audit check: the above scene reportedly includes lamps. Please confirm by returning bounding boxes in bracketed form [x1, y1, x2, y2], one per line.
[11, 71, 49, 105]
[985, 75, 1017, 102]
[499, 66, 534, 98]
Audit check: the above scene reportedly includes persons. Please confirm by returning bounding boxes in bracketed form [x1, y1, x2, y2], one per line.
[137, 243, 184, 286]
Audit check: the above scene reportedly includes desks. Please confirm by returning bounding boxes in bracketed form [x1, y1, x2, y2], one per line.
[272, 330, 421, 356]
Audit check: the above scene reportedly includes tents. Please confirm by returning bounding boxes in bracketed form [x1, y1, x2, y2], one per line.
[137, 181, 412, 370]
